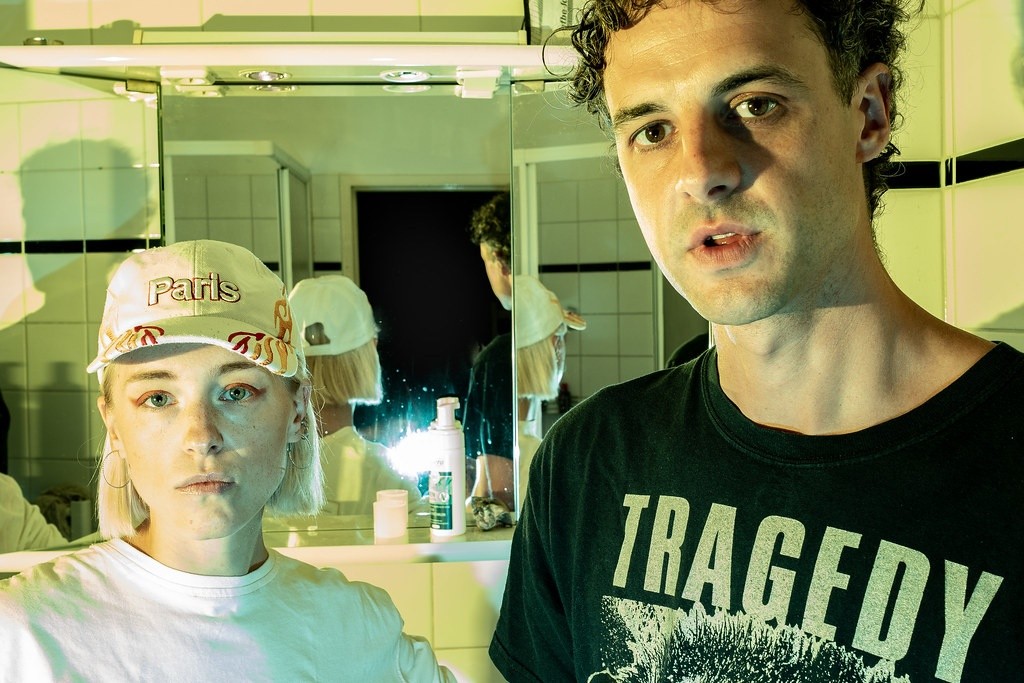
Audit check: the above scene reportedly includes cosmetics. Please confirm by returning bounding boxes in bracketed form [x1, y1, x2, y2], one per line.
[428, 398, 465, 537]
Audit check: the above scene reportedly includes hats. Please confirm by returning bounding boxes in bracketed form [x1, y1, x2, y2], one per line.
[86, 241, 306, 386]
[514, 274, 586, 349]
[287, 275, 379, 358]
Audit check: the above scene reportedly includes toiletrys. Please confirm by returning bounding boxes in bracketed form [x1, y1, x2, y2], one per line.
[426, 398, 468, 539]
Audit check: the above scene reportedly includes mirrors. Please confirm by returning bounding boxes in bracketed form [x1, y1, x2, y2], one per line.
[161, 82, 663, 515]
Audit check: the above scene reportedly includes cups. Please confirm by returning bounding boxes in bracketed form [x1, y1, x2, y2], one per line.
[376, 489, 408, 527]
[373, 501, 405, 538]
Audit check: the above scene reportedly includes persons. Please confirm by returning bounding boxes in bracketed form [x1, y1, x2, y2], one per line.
[514, 273, 587, 514]
[490, 0, 1024, 683]
[463, 194, 513, 507]
[273, 275, 424, 516]
[0, 472, 69, 554]
[0, 241, 458, 683]
